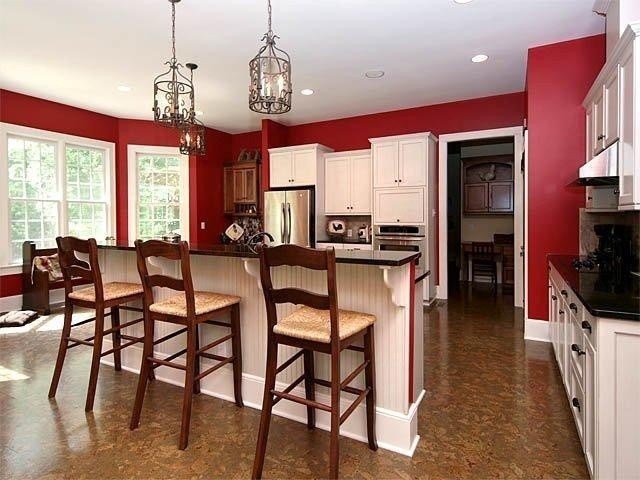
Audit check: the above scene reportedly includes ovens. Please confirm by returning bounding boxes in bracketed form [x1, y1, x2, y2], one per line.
[372, 226, 426, 274]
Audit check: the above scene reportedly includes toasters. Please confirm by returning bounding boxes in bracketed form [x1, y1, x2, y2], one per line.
[359, 240, 369, 243]
[358, 223, 368, 239]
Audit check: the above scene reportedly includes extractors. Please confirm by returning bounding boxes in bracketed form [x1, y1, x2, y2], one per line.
[566, 141, 619, 186]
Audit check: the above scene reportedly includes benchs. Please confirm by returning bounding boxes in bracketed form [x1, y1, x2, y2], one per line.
[22, 241, 94, 316]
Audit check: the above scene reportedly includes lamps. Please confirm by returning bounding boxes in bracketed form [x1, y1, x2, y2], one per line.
[178, 63, 207, 157]
[248, 0, 293, 114]
[153, 0, 195, 128]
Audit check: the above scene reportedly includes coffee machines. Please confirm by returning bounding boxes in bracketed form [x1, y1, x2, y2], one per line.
[593, 223, 634, 292]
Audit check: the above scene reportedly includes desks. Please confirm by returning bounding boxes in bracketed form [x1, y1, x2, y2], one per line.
[462, 241, 514, 284]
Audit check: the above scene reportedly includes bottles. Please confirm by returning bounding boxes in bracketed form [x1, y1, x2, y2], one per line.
[105, 236, 115, 247]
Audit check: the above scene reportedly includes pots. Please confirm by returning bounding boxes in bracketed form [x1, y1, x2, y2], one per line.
[162, 232, 181, 243]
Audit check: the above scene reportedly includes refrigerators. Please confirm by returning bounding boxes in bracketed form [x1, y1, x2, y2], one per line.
[264, 190, 312, 247]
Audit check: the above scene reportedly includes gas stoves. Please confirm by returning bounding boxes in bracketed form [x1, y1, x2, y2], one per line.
[572, 256, 599, 272]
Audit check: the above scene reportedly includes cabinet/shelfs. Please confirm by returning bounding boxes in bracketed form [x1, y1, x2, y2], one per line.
[586, 63, 619, 164]
[264, 131, 439, 250]
[223, 159, 263, 216]
[549, 257, 640, 480]
[463, 161, 513, 218]
[618, 39, 640, 212]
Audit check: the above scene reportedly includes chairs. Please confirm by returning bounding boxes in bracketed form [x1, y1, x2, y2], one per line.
[472, 241, 497, 284]
